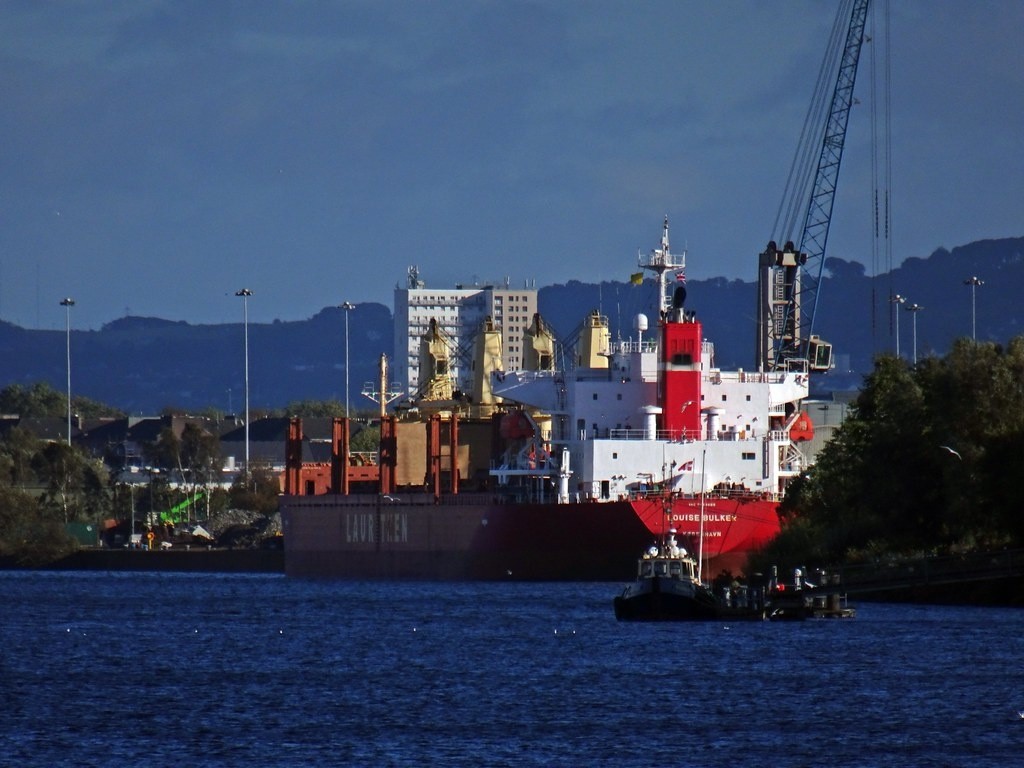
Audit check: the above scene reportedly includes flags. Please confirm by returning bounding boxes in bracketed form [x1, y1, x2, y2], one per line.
[631, 272, 644, 287]
[675, 271, 687, 285]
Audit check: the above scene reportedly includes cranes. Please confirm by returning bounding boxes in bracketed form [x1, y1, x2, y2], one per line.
[751, 0, 891, 375]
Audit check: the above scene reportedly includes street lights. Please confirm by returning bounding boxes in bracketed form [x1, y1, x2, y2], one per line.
[338, 301, 356, 419]
[235, 288, 252, 484]
[59, 297, 75, 492]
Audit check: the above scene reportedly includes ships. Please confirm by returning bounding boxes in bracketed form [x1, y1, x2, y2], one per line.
[278, 214, 855, 583]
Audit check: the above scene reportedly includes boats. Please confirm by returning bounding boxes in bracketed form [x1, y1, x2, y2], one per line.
[607, 450, 856, 623]
